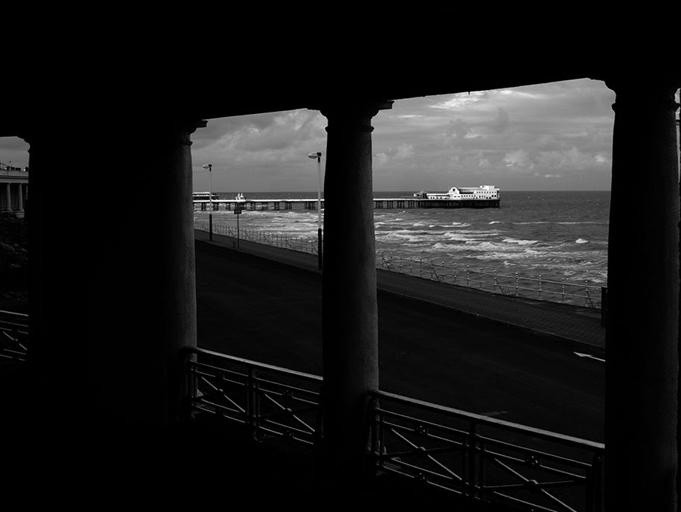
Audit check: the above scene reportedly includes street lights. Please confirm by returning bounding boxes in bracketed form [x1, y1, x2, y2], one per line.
[308, 152, 323, 272]
[203, 163, 214, 242]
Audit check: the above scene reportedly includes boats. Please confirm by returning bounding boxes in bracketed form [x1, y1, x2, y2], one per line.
[193, 192, 220, 200]
